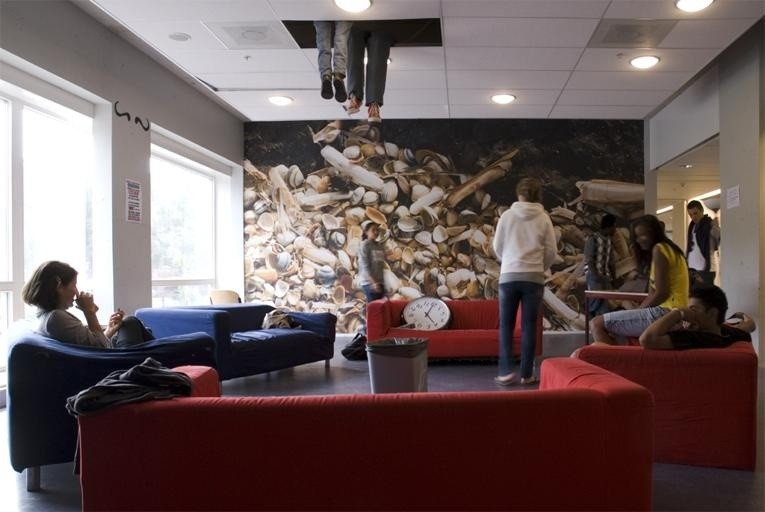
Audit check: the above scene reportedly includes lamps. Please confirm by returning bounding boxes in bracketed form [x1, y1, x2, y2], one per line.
[629, 54, 660, 70]
[269, 95, 293, 110]
[491, 93, 516, 106]
[332, 0, 373, 13]
[674, 0, 716, 15]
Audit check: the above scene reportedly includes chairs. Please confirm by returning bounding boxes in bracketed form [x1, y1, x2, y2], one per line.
[208, 288, 244, 305]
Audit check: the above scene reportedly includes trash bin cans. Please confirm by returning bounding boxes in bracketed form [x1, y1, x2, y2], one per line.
[365, 337, 429, 394]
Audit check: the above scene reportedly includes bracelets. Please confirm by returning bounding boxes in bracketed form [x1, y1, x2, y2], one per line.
[671, 308, 686, 320]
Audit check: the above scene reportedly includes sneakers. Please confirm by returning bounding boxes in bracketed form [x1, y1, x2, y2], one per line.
[334, 76, 347, 101]
[368, 102, 381, 122]
[348, 97, 362, 112]
[321, 76, 333, 99]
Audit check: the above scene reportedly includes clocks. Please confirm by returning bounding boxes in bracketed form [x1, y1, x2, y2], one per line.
[399, 295, 456, 333]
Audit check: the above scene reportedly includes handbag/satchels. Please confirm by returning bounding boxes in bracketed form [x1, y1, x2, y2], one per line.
[341, 333, 367, 360]
[262, 310, 290, 330]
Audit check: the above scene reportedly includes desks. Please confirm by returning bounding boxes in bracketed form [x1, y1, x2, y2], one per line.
[541, 324, 608, 359]
[585, 290, 649, 345]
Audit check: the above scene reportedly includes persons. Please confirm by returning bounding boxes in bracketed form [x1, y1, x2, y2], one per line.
[313, 21, 352, 102]
[20, 260, 153, 353]
[684, 200, 720, 286]
[583, 214, 618, 320]
[592, 215, 692, 349]
[492, 176, 557, 385]
[356, 221, 388, 337]
[342, 21, 390, 121]
[570, 284, 757, 358]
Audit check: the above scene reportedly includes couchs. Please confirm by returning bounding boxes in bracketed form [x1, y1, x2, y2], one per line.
[568, 322, 759, 471]
[5, 326, 221, 493]
[363, 291, 626, 364]
[69, 355, 659, 512]
[132, 300, 342, 393]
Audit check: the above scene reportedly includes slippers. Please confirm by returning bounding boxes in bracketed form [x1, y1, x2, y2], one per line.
[494, 376, 512, 386]
[526, 377, 538, 385]
